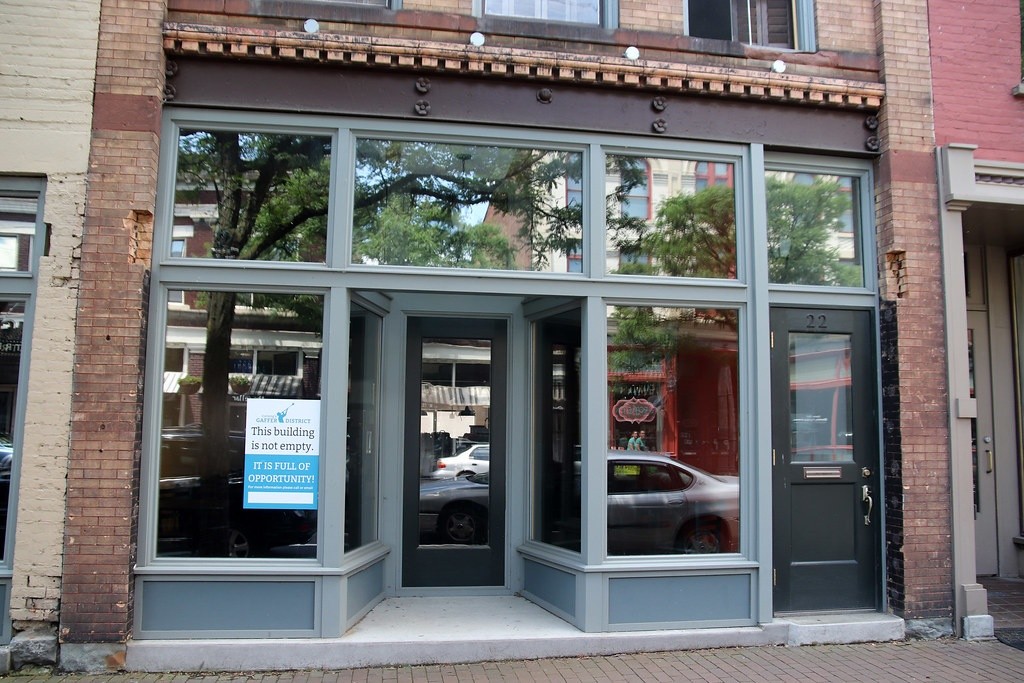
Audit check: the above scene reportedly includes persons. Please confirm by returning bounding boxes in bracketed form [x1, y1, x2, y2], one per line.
[626, 429, 651, 452]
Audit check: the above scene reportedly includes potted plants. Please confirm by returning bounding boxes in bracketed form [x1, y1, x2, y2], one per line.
[229, 375, 254, 394]
[178, 374, 202, 395]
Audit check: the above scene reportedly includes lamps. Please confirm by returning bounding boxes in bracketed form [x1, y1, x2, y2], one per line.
[421, 410, 427, 415]
[449, 405, 455, 419]
[457, 406, 477, 417]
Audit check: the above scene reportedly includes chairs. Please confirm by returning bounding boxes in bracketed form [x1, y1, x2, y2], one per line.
[647, 472, 673, 491]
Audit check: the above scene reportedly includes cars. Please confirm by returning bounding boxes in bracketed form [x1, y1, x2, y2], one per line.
[412, 472, 494, 546]
[430, 442, 493, 481]
[608, 450, 741, 557]
[159, 424, 318, 557]
